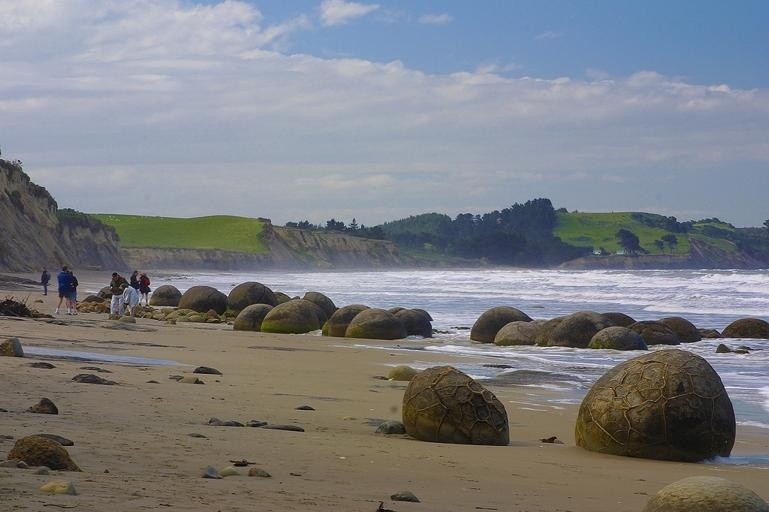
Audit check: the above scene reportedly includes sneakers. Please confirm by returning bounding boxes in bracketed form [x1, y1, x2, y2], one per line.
[43, 293, 47, 295]
[54, 311, 61, 315]
[67, 310, 79, 315]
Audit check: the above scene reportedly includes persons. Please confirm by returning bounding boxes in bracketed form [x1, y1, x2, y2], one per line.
[119, 283, 139, 319]
[55, 265, 73, 316]
[109, 272, 129, 319]
[66, 270, 79, 315]
[138, 273, 151, 306]
[39, 266, 51, 295]
[130, 270, 140, 297]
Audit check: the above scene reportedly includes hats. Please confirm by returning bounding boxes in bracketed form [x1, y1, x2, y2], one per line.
[112, 272, 118, 277]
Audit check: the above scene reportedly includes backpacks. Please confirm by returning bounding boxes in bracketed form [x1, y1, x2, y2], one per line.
[45, 272, 51, 280]
[142, 277, 150, 287]
[68, 278, 78, 292]
